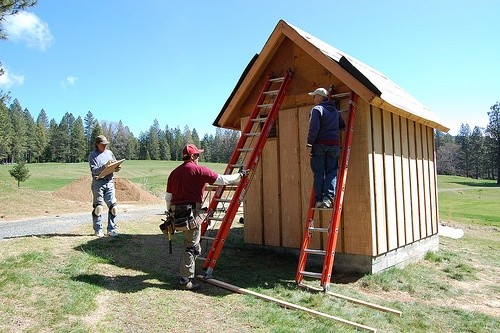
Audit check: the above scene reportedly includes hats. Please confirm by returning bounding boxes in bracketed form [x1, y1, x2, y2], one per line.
[183, 144, 204, 158]
[96, 135, 109, 145]
[308, 88, 329, 98]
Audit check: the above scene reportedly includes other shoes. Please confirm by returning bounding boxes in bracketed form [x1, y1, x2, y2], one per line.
[178, 281, 200, 290]
[316, 202, 324, 209]
[322, 199, 333, 208]
[107, 232, 119, 237]
[95, 232, 104, 238]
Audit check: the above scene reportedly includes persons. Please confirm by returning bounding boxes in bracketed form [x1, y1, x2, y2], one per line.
[88, 135, 120, 237]
[166, 144, 245, 289]
[307, 87, 345, 208]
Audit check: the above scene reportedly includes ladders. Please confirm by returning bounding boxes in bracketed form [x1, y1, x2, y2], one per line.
[202, 68, 294, 279]
[295, 84, 356, 293]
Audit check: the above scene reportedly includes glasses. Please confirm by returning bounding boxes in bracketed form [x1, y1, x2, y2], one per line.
[313, 96, 322, 99]
[193, 154, 199, 157]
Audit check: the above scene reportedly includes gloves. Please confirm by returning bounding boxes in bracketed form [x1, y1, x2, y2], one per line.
[238, 165, 250, 178]
